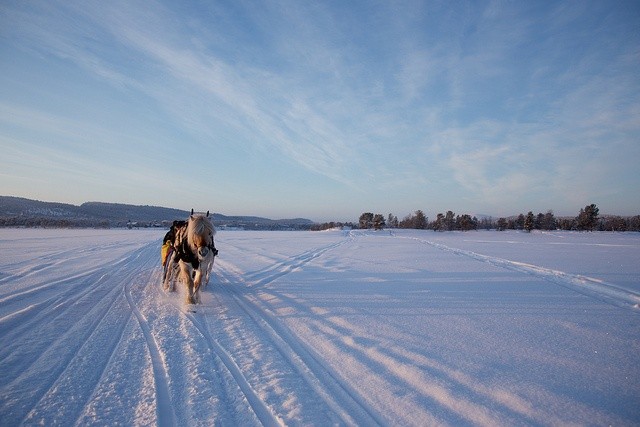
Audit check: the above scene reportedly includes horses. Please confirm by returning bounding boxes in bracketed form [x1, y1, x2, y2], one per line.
[163, 214, 212, 312]
